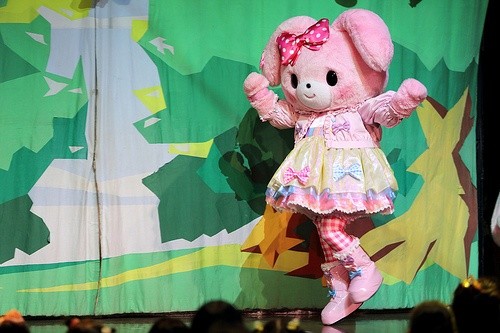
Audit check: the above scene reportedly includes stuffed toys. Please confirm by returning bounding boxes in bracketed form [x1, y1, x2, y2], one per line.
[244, 8, 428, 324]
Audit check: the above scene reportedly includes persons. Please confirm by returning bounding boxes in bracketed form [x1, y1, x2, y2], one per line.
[0, 190, 500, 333]
